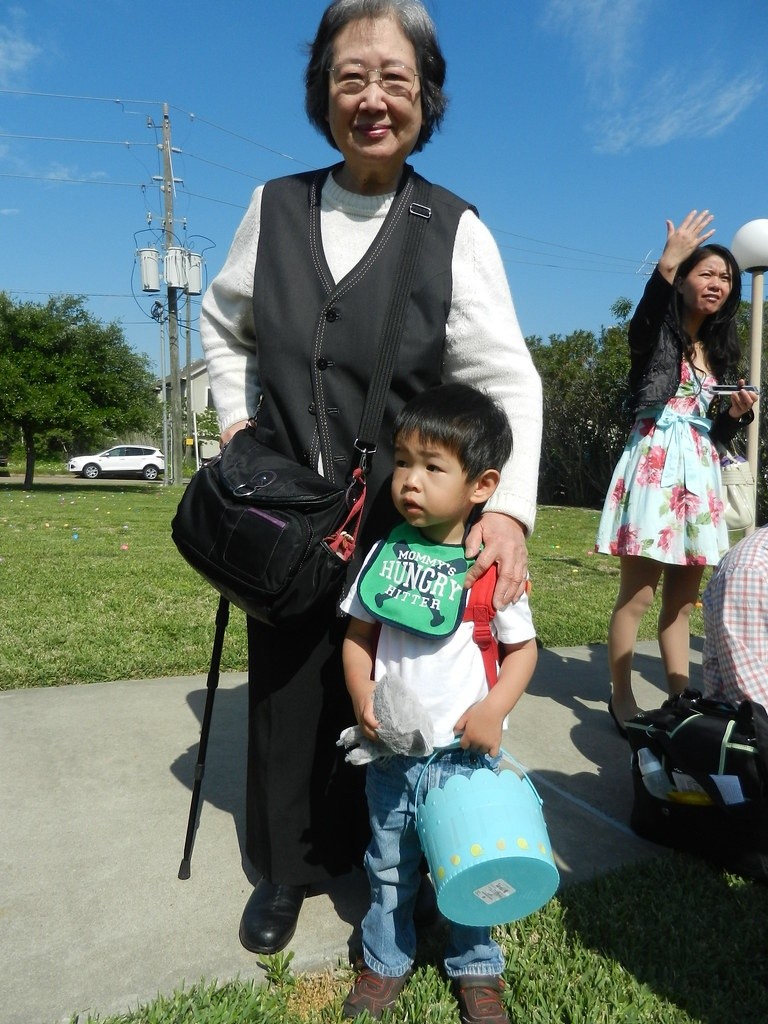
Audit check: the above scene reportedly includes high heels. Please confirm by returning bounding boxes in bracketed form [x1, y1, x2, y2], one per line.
[608, 695, 637, 739]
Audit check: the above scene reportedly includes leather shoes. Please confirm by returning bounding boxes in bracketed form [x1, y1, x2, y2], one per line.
[239, 873, 308, 955]
[301, 833, 370, 873]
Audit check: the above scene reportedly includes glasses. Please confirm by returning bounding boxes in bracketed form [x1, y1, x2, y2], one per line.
[326, 63, 420, 96]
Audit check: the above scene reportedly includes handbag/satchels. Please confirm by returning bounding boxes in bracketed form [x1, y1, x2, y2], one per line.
[171, 429, 372, 630]
[627, 689, 768, 856]
[712, 436, 755, 532]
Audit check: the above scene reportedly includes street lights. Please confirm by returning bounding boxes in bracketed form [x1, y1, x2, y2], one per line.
[728, 217, 768, 543]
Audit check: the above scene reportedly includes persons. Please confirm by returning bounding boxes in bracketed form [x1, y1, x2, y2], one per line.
[702, 523, 768, 718]
[201, 1, 544, 952]
[343, 382, 539, 1024]
[594, 208, 759, 738]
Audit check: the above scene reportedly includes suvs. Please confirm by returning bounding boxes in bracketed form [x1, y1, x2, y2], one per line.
[66, 444, 166, 480]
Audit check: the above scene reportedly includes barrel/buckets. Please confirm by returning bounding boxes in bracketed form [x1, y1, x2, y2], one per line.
[412, 739, 560, 927]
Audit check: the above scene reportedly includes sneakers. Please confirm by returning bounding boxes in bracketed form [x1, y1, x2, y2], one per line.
[342, 959, 413, 1021]
[453, 974, 509, 1024]
